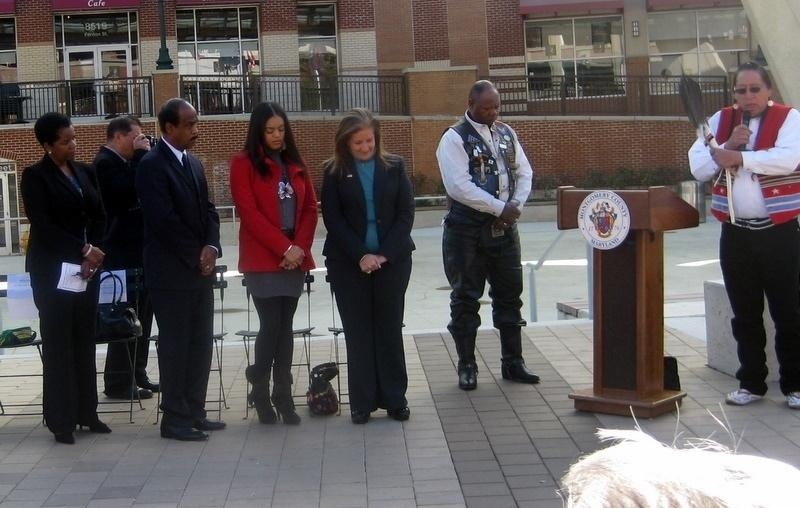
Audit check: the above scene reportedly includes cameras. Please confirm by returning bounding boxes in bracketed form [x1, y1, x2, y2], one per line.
[144, 135, 158, 148]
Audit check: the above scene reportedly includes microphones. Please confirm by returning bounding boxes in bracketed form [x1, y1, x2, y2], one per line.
[740, 110, 751, 151]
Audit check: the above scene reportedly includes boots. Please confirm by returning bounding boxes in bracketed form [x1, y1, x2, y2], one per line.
[248, 359, 300, 425]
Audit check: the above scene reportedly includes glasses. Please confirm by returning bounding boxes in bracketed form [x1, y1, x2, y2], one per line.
[733, 86, 763, 95]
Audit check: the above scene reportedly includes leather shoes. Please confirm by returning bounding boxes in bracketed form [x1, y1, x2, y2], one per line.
[120, 376, 161, 399]
[387, 403, 411, 420]
[457, 360, 477, 389]
[350, 409, 369, 423]
[161, 419, 225, 441]
[501, 357, 540, 382]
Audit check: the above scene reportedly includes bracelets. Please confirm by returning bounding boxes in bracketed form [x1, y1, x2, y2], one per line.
[83, 244, 95, 258]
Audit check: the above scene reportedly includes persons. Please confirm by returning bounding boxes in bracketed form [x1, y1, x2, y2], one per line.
[89, 114, 162, 397]
[16, 111, 114, 440]
[228, 101, 321, 427]
[436, 77, 541, 390]
[137, 98, 227, 443]
[686, 58, 800, 411]
[318, 108, 417, 422]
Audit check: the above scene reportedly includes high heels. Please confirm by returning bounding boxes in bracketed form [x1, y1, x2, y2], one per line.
[55, 427, 74, 444]
[80, 418, 111, 433]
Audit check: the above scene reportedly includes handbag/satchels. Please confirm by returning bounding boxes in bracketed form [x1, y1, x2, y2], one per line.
[306, 371, 338, 414]
[95, 305, 143, 342]
[0, 326, 36, 345]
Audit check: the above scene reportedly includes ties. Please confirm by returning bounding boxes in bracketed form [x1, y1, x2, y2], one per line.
[182, 153, 198, 196]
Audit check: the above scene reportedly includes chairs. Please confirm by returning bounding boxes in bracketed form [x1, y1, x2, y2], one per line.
[325, 259, 405, 416]
[95, 268, 145, 423]
[0, 275, 48, 425]
[147, 265, 230, 425]
[235, 269, 315, 419]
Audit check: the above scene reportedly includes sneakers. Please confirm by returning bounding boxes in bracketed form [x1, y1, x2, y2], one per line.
[726, 388, 761, 405]
[787, 392, 800, 407]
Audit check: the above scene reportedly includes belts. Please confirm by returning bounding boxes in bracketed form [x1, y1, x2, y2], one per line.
[730, 217, 772, 230]
